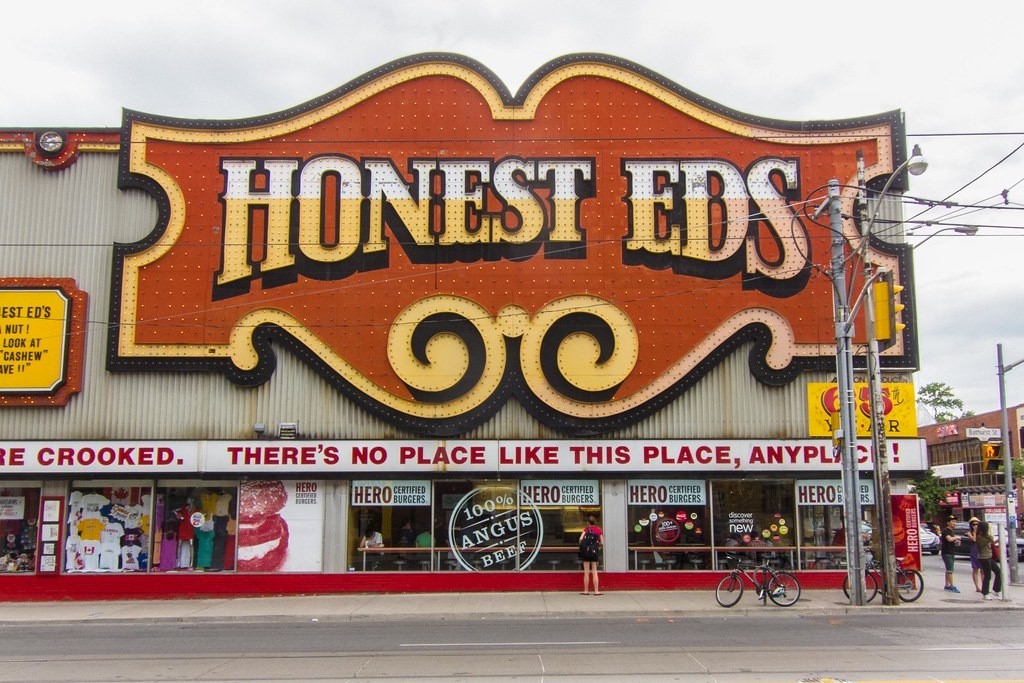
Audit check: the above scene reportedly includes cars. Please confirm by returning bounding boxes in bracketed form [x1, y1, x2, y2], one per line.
[953, 521, 1024, 561]
[919, 526, 942, 555]
[920, 521, 938, 535]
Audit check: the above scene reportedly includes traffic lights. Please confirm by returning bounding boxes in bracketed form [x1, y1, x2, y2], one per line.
[889, 268, 906, 337]
[982, 443, 1004, 472]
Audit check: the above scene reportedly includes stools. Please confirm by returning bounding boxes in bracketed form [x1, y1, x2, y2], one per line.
[368, 559, 845, 571]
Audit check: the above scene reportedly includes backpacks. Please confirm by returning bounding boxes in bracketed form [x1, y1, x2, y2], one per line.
[580, 527, 599, 558]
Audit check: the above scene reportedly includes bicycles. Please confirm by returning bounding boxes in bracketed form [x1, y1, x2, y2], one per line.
[716, 554, 802, 608]
[843, 547, 925, 603]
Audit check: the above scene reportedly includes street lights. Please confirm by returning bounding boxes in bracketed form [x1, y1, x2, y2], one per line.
[825, 143, 930, 602]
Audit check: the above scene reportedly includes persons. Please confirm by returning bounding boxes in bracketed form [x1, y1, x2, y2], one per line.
[398, 518, 446, 560]
[941, 516, 962, 593]
[976, 521, 1001, 600]
[967, 517, 984, 592]
[579, 516, 604, 595]
[725, 531, 775, 566]
[360, 529, 384, 562]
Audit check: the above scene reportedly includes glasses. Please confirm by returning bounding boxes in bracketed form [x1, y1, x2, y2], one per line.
[972, 524, 978, 526]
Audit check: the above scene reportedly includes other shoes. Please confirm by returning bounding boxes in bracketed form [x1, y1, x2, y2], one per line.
[949, 586, 960, 593]
[983, 595, 992, 600]
[944, 585, 951, 589]
[992, 590, 1000, 600]
[976, 590, 982, 592]
[989, 592, 991, 593]
[594, 592, 603, 595]
[580, 592, 589, 595]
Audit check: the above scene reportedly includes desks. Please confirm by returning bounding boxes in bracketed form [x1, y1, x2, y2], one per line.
[357, 547, 871, 572]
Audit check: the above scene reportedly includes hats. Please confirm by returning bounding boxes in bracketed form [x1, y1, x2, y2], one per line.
[945, 515, 958, 522]
[969, 517, 981, 522]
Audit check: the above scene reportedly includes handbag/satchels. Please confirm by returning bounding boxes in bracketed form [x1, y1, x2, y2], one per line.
[991, 540, 1000, 562]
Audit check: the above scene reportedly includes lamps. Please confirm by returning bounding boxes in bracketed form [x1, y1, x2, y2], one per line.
[254, 424, 265, 439]
